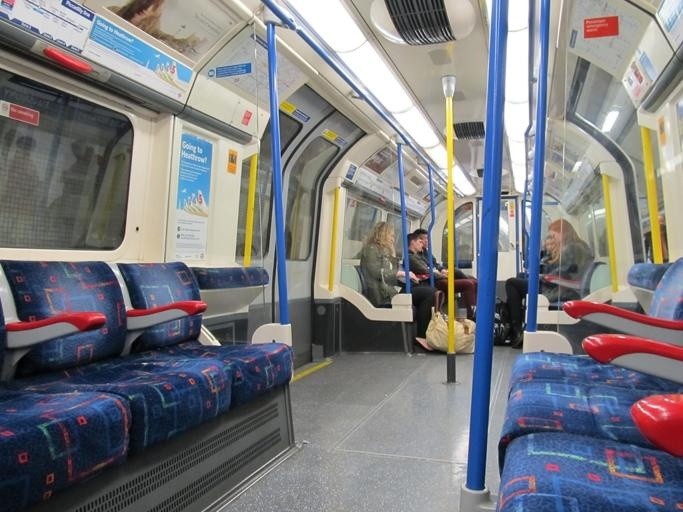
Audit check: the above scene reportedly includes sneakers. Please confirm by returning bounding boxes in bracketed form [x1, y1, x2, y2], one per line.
[416, 337, 434, 351]
[512, 325, 523, 349]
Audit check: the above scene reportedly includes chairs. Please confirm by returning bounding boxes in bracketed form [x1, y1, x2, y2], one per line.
[498, 394, 683, 511]
[506, 256, 683, 399]
[341, 257, 439, 354]
[1, 261, 234, 456]
[117, 261, 296, 405]
[1, 303, 133, 512]
[497, 334, 682, 475]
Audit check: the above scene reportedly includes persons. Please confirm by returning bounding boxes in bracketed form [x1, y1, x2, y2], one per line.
[412, 228, 468, 279]
[402, 232, 477, 321]
[358, 220, 436, 353]
[503, 217, 594, 348]
[105, 0, 207, 56]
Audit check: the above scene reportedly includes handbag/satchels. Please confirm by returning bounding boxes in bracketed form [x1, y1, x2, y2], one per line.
[426, 311, 476, 354]
[494, 301, 510, 345]
[382, 284, 399, 302]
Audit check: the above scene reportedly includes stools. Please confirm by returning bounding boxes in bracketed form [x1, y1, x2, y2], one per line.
[189, 265, 270, 319]
[627, 264, 667, 313]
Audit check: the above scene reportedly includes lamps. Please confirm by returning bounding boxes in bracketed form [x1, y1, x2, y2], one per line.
[485, 1, 533, 193]
[287, 1, 476, 197]
